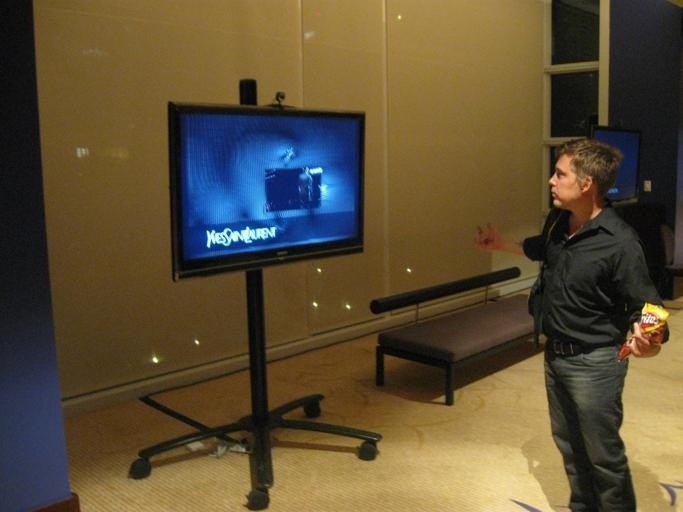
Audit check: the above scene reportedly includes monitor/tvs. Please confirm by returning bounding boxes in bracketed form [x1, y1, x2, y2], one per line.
[167, 100, 367, 281]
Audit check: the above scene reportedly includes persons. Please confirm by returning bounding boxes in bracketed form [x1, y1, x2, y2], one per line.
[474, 136, 671, 511]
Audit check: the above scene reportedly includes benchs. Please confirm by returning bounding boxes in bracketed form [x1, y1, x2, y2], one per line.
[370, 267, 535, 406]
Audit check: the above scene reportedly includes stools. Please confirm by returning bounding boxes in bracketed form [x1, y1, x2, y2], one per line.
[666, 264, 683, 301]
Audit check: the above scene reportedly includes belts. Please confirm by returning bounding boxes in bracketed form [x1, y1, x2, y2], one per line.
[553, 339, 584, 356]
